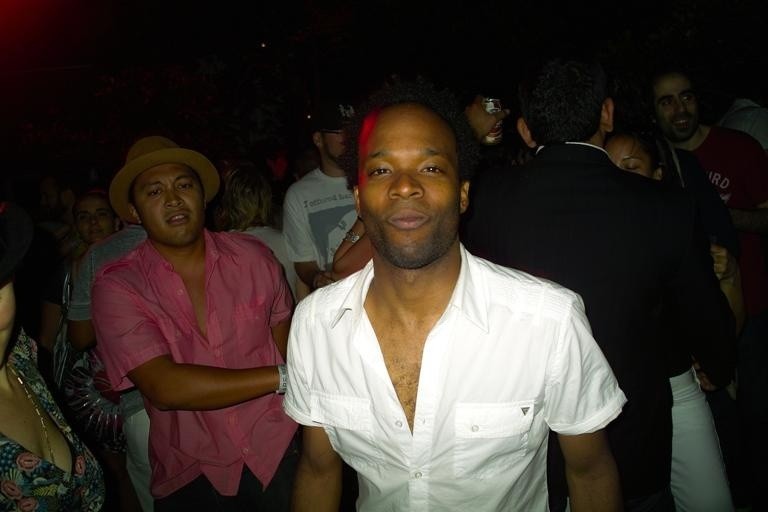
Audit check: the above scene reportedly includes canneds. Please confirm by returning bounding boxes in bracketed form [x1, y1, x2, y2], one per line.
[481, 99, 504, 145]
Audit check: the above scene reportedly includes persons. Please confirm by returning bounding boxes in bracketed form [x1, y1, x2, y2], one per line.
[1, 65, 768, 511]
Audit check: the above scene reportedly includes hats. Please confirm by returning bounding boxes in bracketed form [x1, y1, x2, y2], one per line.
[0, 200, 33, 281]
[108, 136, 222, 227]
